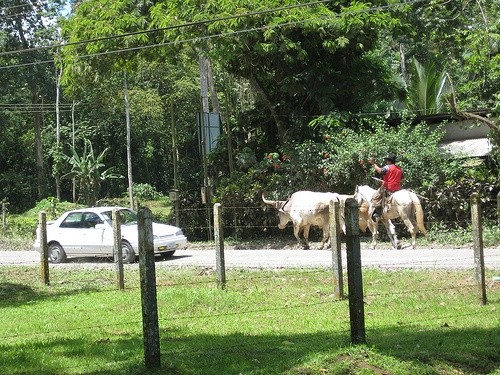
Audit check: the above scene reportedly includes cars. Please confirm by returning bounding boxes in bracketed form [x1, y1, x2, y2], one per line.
[35, 206, 187, 264]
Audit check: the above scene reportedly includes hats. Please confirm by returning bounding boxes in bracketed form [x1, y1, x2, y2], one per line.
[382, 154, 395, 161]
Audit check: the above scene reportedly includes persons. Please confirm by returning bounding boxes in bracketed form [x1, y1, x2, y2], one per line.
[367, 155, 404, 220]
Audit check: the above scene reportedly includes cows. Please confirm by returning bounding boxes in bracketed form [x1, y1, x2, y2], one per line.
[354, 184, 427, 250]
[261, 191, 358, 250]
